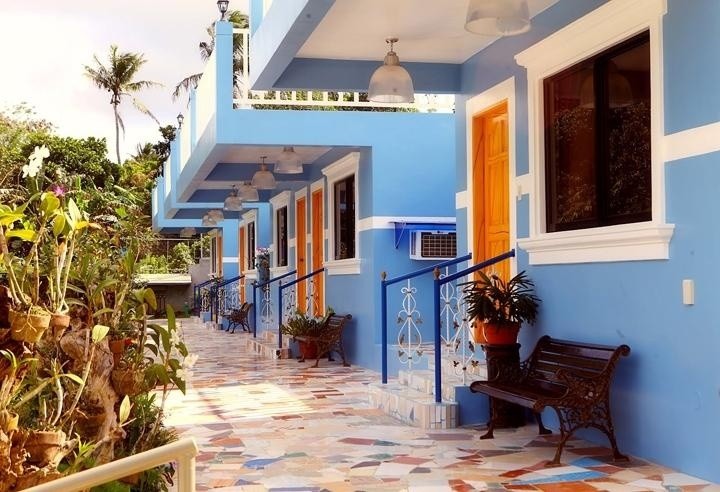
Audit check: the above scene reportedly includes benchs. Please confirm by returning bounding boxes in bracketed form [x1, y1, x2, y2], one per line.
[294, 312, 352, 368]
[469, 334, 631, 467]
[220, 302, 253, 334]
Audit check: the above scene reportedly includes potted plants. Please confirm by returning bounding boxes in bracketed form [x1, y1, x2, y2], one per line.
[455, 269, 542, 345]
[0, 175, 199, 482]
[279, 305, 335, 359]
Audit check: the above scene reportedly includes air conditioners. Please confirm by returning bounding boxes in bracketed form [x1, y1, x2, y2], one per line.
[409, 229, 457, 261]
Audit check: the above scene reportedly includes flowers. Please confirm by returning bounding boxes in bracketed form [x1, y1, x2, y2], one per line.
[257, 246, 272, 256]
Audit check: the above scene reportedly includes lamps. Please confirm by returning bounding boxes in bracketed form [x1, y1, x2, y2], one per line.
[251, 156, 277, 190]
[236, 182, 260, 202]
[208, 209, 224, 221]
[183, 227, 198, 236]
[158, 165, 163, 177]
[177, 112, 184, 129]
[217, 0, 230, 21]
[273, 147, 304, 175]
[180, 229, 193, 238]
[367, 38, 414, 103]
[201, 214, 217, 226]
[464, 0, 531, 36]
[223, 185, 243, 211]
